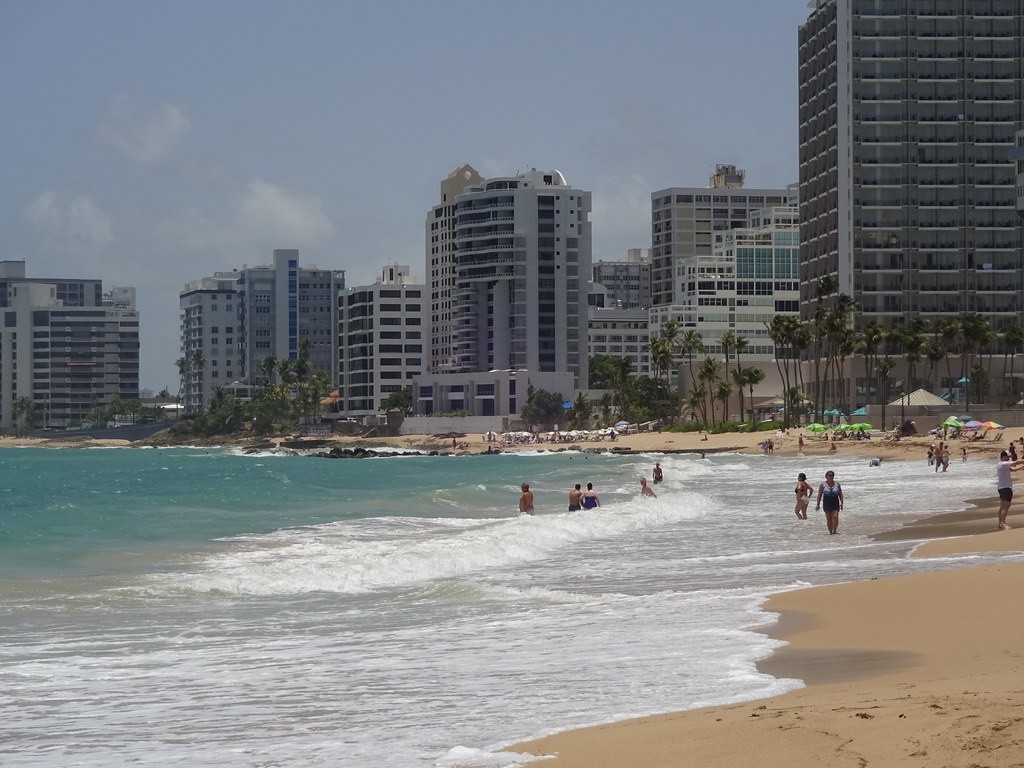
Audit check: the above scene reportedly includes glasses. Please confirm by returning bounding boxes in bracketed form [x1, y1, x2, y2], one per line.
[829, 475, 833, 476]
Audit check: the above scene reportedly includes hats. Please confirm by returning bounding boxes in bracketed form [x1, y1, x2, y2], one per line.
[1001, 451, 1011, 456]
[932, 444, 934, 446]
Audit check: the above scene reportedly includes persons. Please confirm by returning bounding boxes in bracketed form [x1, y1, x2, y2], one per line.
[653, 463, 662, 484]
[701, 449, 705, 458]
[762, 439, 774, 455]
[519, 483, 534, 516]
[569, 483, 601, 511]
[822, 422, 902, 451]
[640, 478, 658, 498]
[997, 437, 1024, 529]
[481, 429, 619, 444]
[815, 471, 843, 535]
[943, 424, 984, 441]
[701, 435, 707, 441]
[453, 437, 457, 450]
[927, 442, 951, 472]
[798, 433, 804, 451]
[795, 473, 813, 519]
[962, 448, 967, 461]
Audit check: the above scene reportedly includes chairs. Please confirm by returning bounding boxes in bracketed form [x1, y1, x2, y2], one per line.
[806, 427, 1003, 442]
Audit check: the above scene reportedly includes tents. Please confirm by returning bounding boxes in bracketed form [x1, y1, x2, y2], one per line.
[805, 423, 872, 432]
[941, 415, 1004, 430]
[486, 420, 630, 436]
